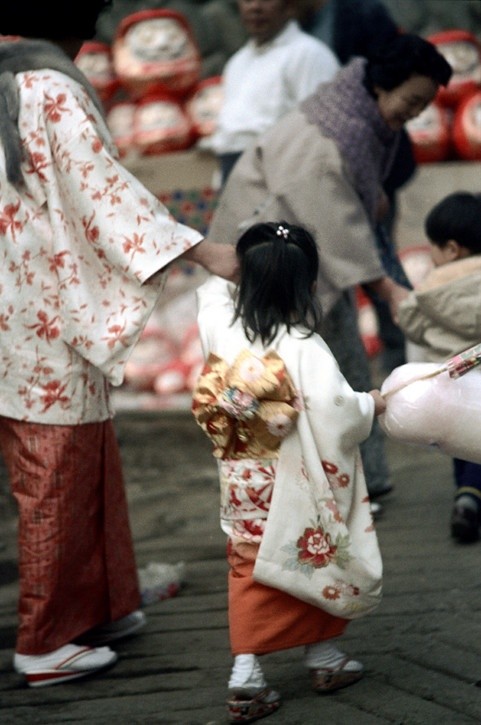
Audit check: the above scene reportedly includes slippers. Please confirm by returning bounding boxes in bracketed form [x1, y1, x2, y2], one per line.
[91, 620, 147, 643]
[225, 686, 280, 723]
[25, 646, 102, 687]
[310, 654, 364, 693]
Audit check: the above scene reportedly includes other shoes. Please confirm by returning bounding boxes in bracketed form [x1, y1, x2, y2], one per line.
[445, 496, 480, 541]
[367, 484, 393, 500]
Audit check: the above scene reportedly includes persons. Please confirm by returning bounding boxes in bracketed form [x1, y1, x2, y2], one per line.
[200, 32, 452, 538]
[396, 189, 481, 545]
[0, 0, 242, 689]
[202, 0, 340, 205]
[183, 218, 392, 724]
